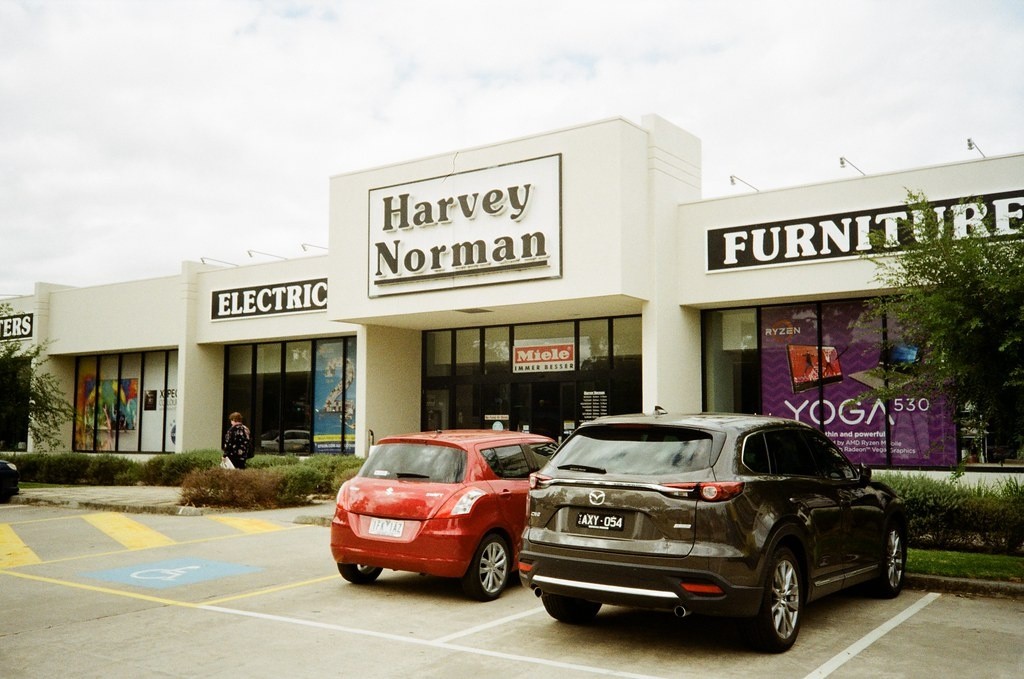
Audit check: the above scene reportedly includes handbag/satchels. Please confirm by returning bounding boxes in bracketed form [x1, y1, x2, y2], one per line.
[219, 456, 236, 469]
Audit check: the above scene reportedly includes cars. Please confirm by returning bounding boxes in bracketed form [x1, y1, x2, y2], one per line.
[330, 429, 560, 602]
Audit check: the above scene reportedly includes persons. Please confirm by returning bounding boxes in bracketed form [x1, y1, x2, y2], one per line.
[222, 412, 250, 469]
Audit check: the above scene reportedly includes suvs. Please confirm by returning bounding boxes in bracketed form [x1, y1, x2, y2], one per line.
[518, 406, 911, 653]
[261, 430, 310, 453]
